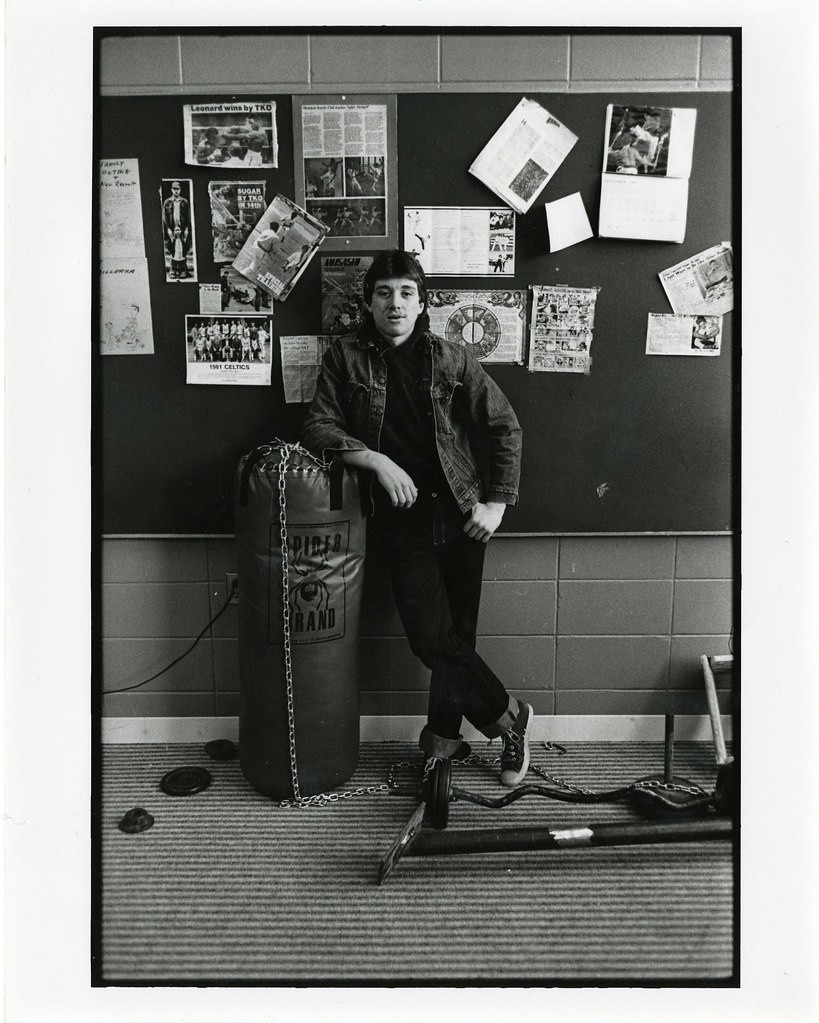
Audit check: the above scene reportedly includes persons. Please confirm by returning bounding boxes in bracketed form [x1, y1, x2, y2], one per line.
[163, 181, 191, 279]
[693, 316, 720, 349]
[306, 157, 385, 236]
[196, 114, 269, 166]
[191, 319, 269, 363]
[241, 211, 310, 277]
[299, 251, 534, 786]
[489, 211, 515, 273]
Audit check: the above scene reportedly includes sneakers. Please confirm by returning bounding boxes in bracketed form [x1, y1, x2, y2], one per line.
[498, 701, 533, 786]
[420, 750, 439, 785]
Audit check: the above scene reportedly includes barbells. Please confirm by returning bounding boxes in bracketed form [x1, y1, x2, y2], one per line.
[386, 755, 741, 830]
